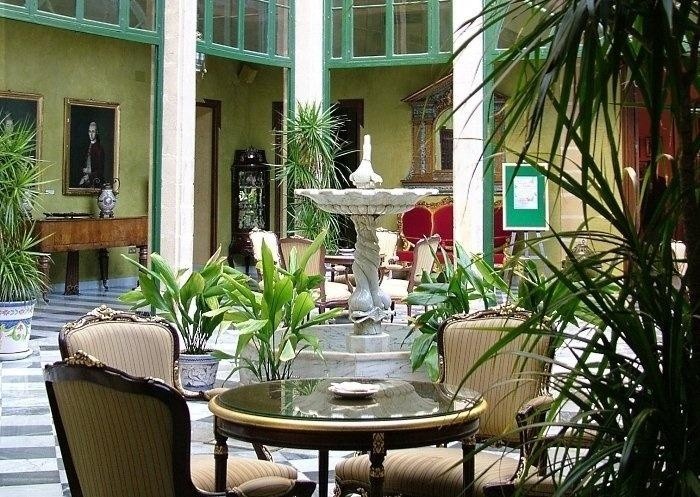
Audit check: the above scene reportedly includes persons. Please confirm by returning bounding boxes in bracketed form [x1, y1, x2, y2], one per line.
[79, 122, 103, 188]
[4, 112, 15, 136]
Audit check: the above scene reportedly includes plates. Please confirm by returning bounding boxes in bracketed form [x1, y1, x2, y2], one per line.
[339, 249, 355, 252]
[328, 382, 379, 398]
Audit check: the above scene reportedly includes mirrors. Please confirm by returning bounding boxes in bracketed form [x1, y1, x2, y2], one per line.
[433, 109, 454, 171]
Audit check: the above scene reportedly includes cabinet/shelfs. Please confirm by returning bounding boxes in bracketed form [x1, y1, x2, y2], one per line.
[228, 149, 274, 268]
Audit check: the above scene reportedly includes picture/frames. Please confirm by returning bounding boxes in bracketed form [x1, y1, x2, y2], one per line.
[61, 96, 120, 197]
[501, 162, 549, 232]
[1, 89, 41, 194]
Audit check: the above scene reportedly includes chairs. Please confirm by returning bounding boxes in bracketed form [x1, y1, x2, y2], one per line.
[278, 234, 352, 314]
[391, 232, 444, 323]
[248, 226, 283, 280]
[594, 465, 609, 469]
[337, 301, 558, 497]
[375, 228, 399, 278]
[60, 305, 297, 491]
[483, 426, 612, 497]
[41, 351, 315, 496]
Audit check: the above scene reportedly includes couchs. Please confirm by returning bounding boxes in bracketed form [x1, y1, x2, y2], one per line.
[395, 197, 525, 284]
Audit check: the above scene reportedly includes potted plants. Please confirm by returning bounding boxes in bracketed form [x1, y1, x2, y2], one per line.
[117, 244, 223, 391]
[0, 109, 59, 360]
